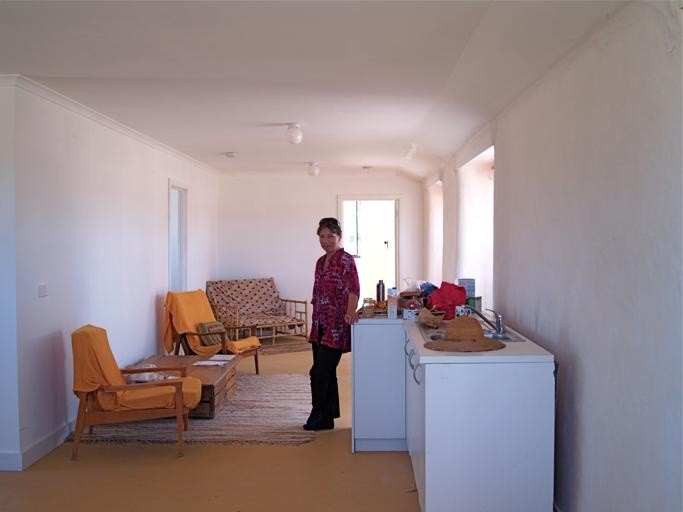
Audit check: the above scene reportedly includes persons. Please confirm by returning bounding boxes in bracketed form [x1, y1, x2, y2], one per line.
[301, 217, 361, 432]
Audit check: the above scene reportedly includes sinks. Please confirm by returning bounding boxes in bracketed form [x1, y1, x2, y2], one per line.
[415, 320, 527, 342]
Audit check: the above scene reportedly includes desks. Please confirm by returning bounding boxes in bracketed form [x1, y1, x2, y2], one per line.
[124, 355, 242, 418]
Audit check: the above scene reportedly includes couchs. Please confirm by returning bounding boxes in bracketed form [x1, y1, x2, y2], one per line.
[206, 277, 310, 344]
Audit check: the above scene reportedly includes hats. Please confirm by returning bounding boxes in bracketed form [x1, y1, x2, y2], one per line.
[424, 318, 505, 352]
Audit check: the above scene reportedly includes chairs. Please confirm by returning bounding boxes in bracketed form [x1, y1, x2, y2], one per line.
[71, 325, 202, 461]
[163, 289, 261, 374]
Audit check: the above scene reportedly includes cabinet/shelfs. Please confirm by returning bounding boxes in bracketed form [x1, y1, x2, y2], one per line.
[351, 319, 406, 452]
[405, 318, 555, 512]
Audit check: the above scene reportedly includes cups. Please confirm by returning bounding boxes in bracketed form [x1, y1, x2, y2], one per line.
[455, 306, 471, 320]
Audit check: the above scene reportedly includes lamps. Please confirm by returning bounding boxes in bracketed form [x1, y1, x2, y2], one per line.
[308, 161, 319, 176]
[286, 124, 302, 144]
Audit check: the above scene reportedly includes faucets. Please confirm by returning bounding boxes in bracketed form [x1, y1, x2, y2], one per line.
[465, 305, 505, 335]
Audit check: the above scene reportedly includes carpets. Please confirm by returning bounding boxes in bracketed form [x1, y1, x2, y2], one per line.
[64, 374, 316, 446]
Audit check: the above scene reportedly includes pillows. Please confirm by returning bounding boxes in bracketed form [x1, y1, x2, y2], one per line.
[198, 322, 229, 345]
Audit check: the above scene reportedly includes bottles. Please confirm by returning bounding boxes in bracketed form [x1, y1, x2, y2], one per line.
[377, 280, 385, 301]
[391, 287, 397, 296]
[363, 298, 374, 317]
